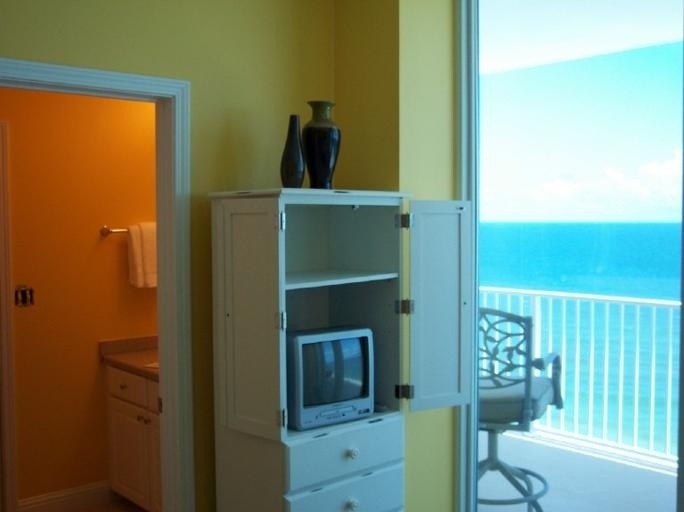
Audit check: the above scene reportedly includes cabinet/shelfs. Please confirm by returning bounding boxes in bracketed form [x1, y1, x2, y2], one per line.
[103, 365, 163, 511]
[211, 186, 472, 511]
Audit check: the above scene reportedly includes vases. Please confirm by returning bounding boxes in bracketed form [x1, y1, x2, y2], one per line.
[280, 114, 306, 189]
[302, 99, 341, 188]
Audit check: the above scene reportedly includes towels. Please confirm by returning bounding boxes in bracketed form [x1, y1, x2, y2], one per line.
[125, 222, 159, 290]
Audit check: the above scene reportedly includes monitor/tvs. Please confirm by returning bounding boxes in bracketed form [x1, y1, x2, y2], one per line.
[288, 325, 377, 428]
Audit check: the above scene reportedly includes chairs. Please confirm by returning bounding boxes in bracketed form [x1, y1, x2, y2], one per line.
[477, 306, 564, 512]
